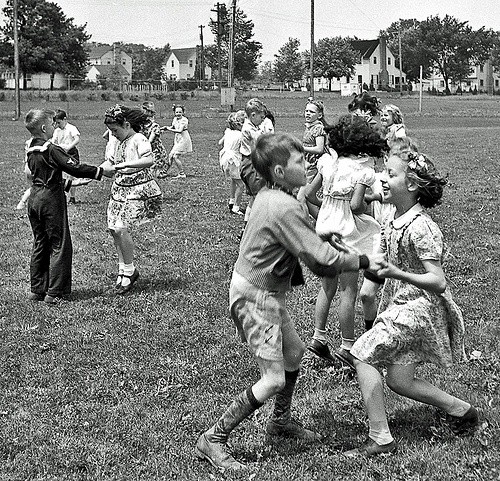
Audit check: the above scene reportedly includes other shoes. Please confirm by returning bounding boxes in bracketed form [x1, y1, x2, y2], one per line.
[172, 173, 187, 178]
[307, 338, 333, 361]
[335, 347, 356, 370]
[44, 295, 63, 305]
[116, 269, 139, 294]
[115, 274, 124, 289]
[231, 209, 245, 216]
[30, 292, 45, 299]
[70, 197, 76, 205]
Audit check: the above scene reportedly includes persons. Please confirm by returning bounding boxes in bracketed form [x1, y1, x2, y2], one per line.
[25, 109, 116, 304]
[159, 105, 194, 177]
[102, 102, 170, 294]
[18, 110, 81, 208]
[195, 91, 479, 472]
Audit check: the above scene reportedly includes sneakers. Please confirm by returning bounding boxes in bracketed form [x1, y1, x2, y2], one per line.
[195, 435, 246, 472]
[268, 417, 324, 440]
[425, 407, 479, 437]
[345, 435, 398, 457]
